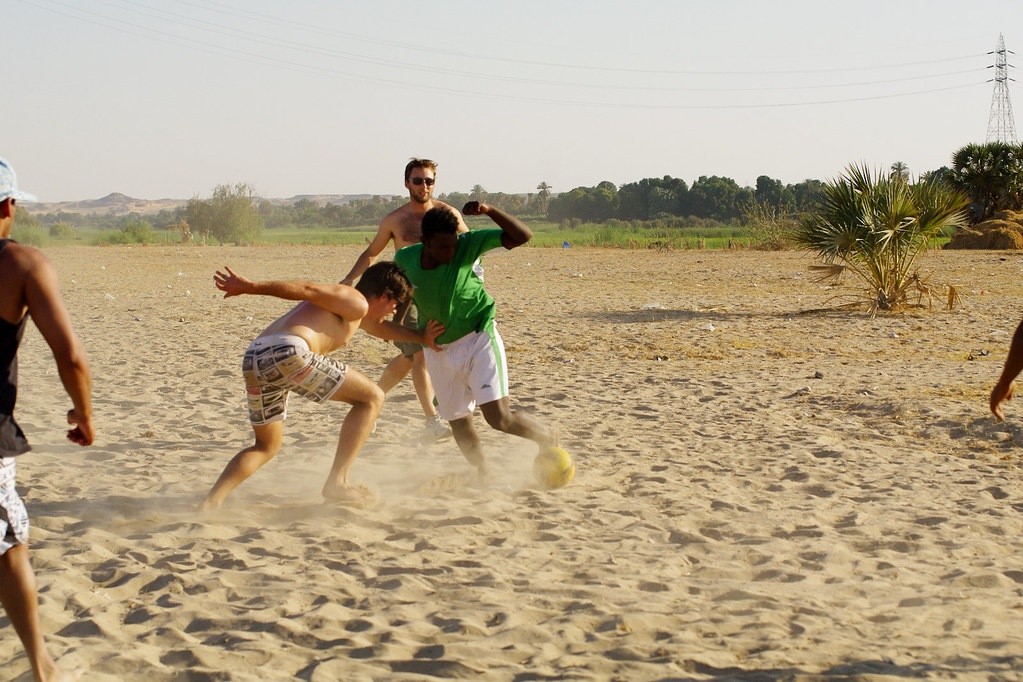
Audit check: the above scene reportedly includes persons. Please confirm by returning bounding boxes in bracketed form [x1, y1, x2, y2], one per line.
[990, 319, 1023, 421]
[338, 156, 472, 441]
[0, 158, 93, 682]
[193, 261, 445, 512]
[385, 200, 559, 477]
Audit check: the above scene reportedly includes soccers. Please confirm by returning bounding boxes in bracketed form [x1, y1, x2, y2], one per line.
[530, 445, 573, 490]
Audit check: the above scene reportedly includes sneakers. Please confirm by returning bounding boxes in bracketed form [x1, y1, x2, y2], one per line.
[370, 421, 377, 434]
[426, 414, 454, 439]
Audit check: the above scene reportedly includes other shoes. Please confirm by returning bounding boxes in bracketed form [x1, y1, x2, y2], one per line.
[538, 427, 560, 454]
[424, 463, 489, 493]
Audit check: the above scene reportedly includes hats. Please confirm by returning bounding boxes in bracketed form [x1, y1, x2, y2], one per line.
[0, 157, 36, 202]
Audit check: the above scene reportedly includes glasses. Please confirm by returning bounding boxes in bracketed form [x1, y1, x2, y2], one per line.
[395, 297, 406, 310]
[408, 177, 435, 186]
